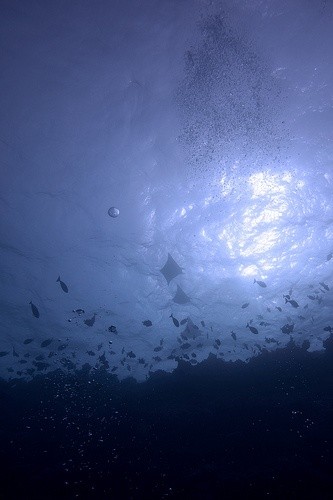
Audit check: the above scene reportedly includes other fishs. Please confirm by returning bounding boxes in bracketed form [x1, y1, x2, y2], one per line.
[56, 276, 68, 293]
[254, 278, 266, 287]
[160, 253, 185, 286]
[0, 282, 333, 379]
